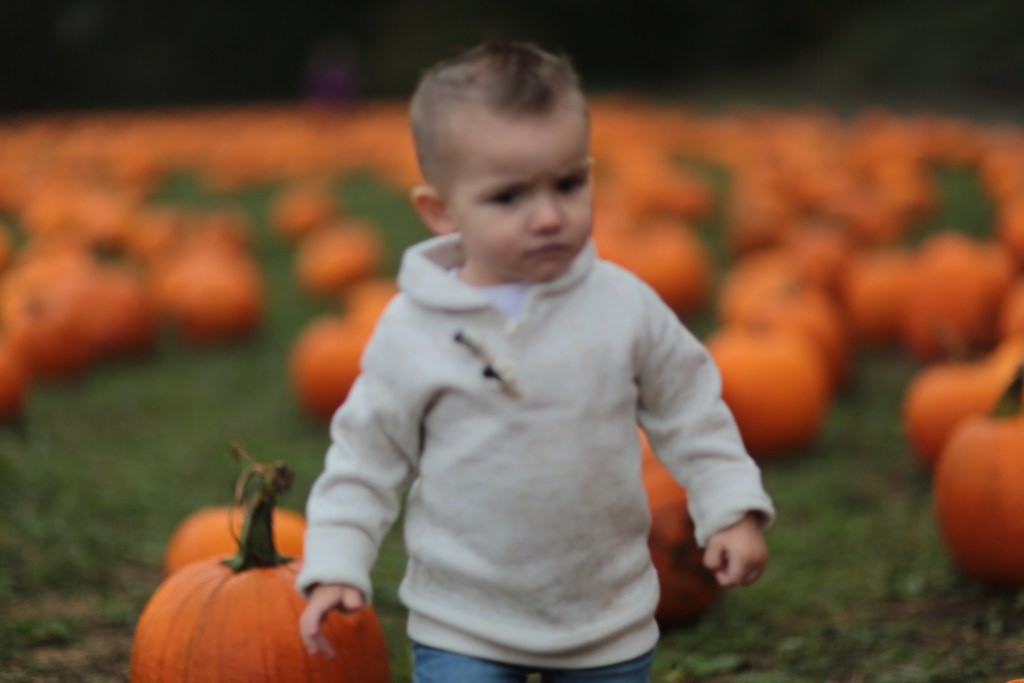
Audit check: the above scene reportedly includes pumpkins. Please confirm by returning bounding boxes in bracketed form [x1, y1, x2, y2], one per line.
[0, 99, 433, 683]
[578, 99, 1023, 630]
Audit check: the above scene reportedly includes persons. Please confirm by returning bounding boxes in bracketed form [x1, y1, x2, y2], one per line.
[297, 41, 778, 683]
